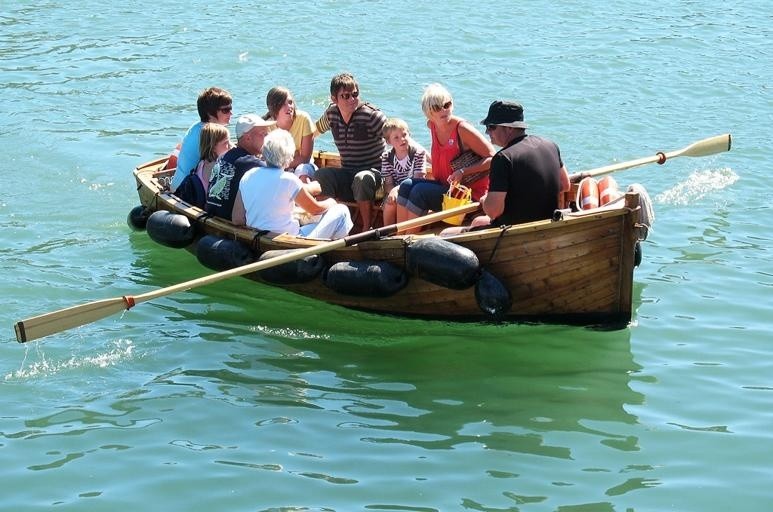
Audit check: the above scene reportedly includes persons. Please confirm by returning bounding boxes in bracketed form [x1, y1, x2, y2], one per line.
[382, 82, 496, 233]
[171, 86, 338, 239]
[311, 73, 431, 231]
[440, 99, 570, 236]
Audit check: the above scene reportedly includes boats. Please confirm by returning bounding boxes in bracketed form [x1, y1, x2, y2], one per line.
[133, 151, 648, 323]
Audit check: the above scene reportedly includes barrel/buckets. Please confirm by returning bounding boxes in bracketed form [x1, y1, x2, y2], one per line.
[441, 184, 472, 226]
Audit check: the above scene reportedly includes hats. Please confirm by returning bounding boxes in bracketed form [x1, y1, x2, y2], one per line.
[479, 99, 529, 129]
[236, 113, 277, 138]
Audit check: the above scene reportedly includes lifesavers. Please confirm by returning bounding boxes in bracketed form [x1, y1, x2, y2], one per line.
[580, 178, 600, 210]
[168, 144, 182, 175]
[598, 176, 617, 208]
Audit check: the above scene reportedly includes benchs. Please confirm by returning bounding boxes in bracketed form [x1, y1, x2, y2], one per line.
[316, 195, 383, 226]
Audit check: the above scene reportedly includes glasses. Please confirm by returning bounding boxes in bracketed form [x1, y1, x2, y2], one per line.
[219, 106, 233, 114]
[485, 125, 497, 131]
[341, 92, 358, 100]
[432, 102, 451, 113]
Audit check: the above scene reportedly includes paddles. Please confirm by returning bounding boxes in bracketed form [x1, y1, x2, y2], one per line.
[14, 135, 732, 343]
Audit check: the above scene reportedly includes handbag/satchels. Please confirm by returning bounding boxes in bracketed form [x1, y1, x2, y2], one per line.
[295, 207, 322, 226]
[448, 120, 488, 186]
[173, 160, 205, 209]
